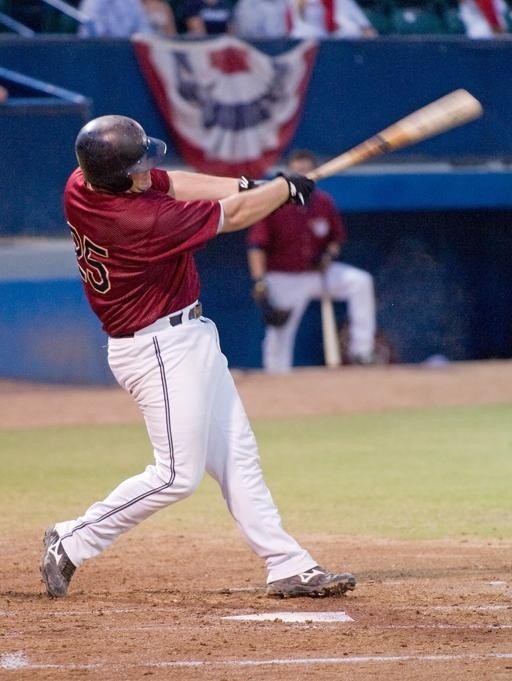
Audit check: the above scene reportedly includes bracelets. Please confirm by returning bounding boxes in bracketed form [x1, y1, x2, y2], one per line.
[251, 277, 263, 285]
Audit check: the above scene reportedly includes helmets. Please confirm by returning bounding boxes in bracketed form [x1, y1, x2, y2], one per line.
[74, 114, 169, 185]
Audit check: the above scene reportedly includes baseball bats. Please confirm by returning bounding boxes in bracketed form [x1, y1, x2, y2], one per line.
[303, 89, 484, 184]
[320, 270, 343, 369]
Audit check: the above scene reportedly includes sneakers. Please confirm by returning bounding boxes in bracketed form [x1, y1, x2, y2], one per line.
[264, 565, 358, 598]
[40, 526, 76, 601]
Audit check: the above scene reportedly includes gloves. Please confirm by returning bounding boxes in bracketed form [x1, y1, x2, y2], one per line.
[237, 169, 315, 216]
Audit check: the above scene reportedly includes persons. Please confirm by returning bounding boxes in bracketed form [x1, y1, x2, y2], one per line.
[78, 1, 381, 40]
[460, 1, 509, 39]
[38, 112, 359, 600]
[245, 150, 380, 371]
